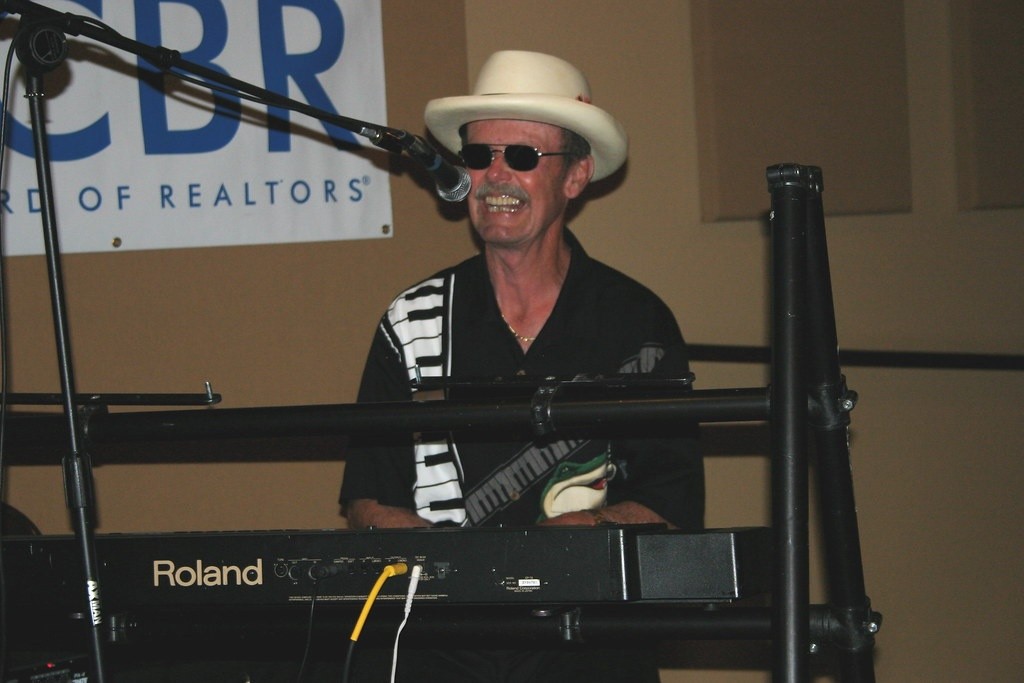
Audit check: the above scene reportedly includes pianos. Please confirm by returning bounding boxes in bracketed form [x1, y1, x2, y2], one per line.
[0, 519, 780, 624]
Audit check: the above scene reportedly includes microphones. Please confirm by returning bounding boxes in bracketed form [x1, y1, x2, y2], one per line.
[399, 129, 472, 202]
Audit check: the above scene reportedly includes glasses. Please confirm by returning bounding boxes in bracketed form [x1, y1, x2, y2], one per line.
[461, 143, 576, 172]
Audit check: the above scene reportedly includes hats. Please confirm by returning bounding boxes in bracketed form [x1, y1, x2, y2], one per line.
[423, 50, 630, 182]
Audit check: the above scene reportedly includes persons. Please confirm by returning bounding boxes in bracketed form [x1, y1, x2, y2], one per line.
[338, 50, 706, 533]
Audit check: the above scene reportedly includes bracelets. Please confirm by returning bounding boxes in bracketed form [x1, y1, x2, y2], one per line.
[582, 507, 608, 524]
